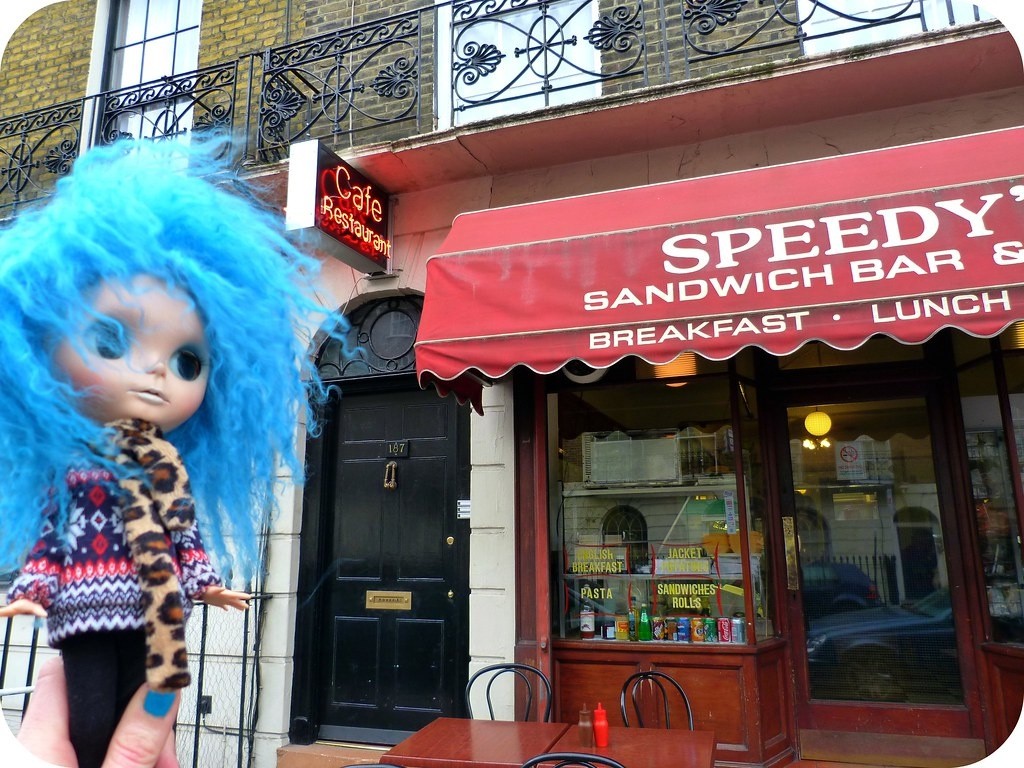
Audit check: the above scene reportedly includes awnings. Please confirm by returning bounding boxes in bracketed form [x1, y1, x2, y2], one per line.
[415, 126, 1024, 416]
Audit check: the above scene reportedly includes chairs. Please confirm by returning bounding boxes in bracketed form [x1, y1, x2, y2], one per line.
[619, 670, 695, 731]
[466, 662, 552, 723]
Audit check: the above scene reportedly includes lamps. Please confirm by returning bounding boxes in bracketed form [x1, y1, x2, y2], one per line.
[804, 405, 833, 437]
[800, 434, 831, 452]
[649, 349, 697, 390]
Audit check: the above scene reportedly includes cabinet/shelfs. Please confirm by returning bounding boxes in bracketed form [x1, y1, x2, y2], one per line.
[558, 479, 768, 641]
[964, 424, 1020, 620]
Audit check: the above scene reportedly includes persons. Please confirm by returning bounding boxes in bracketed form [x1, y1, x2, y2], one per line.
[0, 654, 179, 768]
[0, 137, 366, 768]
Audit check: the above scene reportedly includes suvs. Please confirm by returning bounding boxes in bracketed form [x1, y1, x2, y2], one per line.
[801, 563, 884, 618]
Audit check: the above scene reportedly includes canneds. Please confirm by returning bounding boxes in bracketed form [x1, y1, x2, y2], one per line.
[580, 608, 746, 643]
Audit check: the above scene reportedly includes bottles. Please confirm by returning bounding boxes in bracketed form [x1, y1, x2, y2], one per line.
[578, 595, 653, 642]
[577, 701, 611, 747]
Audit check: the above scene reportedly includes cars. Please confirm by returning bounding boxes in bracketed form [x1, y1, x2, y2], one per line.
[809, 584, 1023, 699]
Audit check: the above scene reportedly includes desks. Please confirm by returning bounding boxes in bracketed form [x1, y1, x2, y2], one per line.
[378, 715, 571, 768]
[536, 722, 716, 768]
